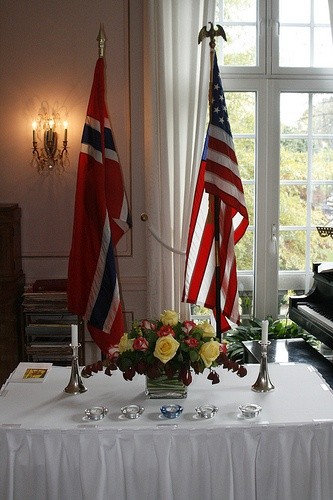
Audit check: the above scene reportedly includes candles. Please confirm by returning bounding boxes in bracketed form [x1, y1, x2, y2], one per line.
[71, 324, 78, 346]
[262, 320, 268, 343]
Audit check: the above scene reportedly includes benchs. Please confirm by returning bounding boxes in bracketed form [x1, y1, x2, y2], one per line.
[241, 339, 332, 390]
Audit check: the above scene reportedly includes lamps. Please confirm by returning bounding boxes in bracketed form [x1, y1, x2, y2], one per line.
[31, 113, 71, 175]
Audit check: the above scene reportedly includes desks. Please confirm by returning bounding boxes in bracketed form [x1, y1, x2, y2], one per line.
[0, 365, 332, 500]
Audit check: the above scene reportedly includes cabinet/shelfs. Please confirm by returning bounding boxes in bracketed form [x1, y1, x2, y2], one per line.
[21, 278, 85, 365]
[0, 203, 24, 388]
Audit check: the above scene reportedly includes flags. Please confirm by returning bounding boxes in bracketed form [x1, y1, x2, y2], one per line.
[66, 57, 132, 358]
[180, 49, 249, 334]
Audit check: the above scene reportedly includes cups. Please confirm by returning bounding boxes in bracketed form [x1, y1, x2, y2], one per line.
[121, 405, 144, 419]
[85, 407, 108, 420]
[195, 404, 218, 419]
[160, 404, 184, 420]
[239, 403, 262, 419]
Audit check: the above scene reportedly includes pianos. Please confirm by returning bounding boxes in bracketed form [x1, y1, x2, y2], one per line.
[288, 262, 333, 352]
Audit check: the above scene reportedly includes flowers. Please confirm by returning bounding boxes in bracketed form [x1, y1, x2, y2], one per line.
[81, 310, 247, 386]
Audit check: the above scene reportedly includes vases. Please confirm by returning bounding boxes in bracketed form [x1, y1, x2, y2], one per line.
[146, 374, 188, 398]
